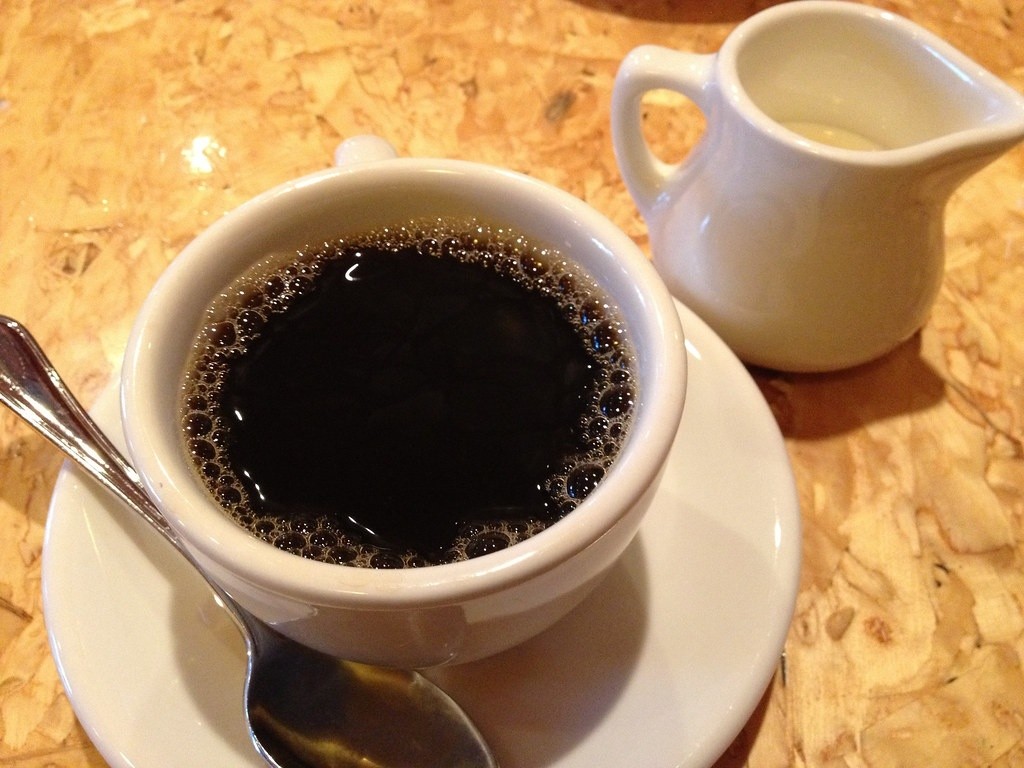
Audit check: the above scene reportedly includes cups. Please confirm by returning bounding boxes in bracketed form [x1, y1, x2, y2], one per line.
[610, 0, 1023, 373]
[120, 135, 689, 665]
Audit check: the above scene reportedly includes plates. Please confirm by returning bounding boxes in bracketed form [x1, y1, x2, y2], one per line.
[41, 293, 800, 767]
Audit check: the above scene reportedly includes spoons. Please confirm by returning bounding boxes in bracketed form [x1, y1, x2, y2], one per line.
[3, 315, 497, 766]
